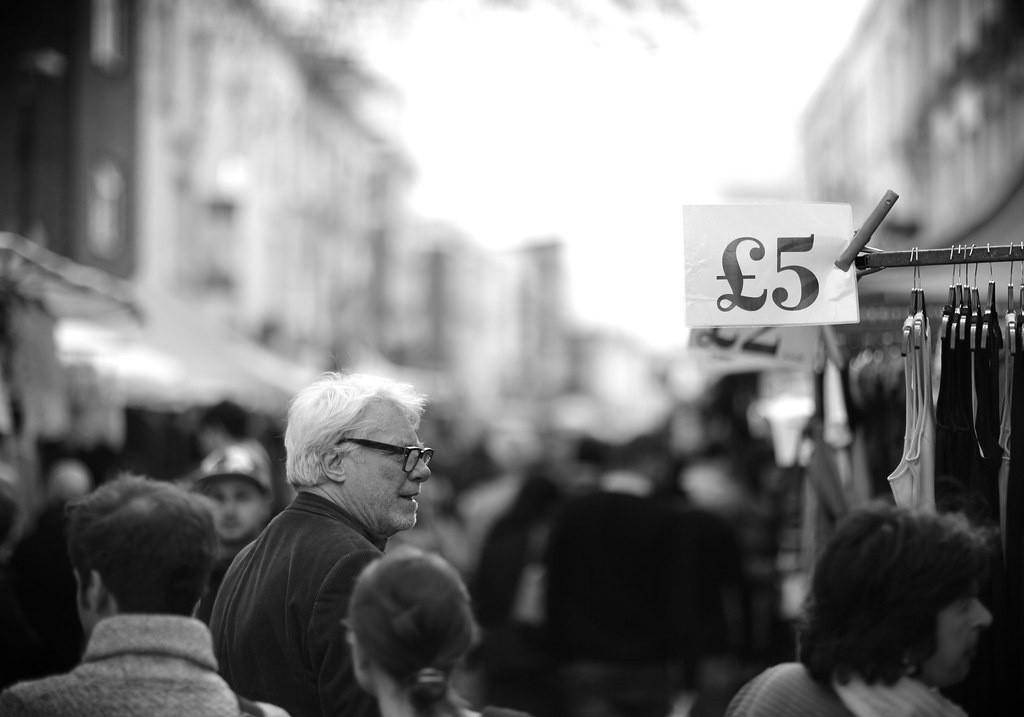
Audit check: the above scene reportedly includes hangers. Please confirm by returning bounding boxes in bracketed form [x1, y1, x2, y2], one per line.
[900, 241, 1024, 357]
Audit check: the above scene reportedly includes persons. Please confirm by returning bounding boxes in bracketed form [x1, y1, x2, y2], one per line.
[721, 494, 994, 717]
[385, 421, 784, 715]
[3, 399, 291, 682]
[0, 474, 289, 717]
[342, 546, 534, 717]
[211, 368, 434, 717]
[185, 442, 279, 623]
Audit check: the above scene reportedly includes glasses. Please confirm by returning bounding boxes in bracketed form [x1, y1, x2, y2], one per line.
[335, 438, 434, 474]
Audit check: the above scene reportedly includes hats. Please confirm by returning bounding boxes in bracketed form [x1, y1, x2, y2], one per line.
[194, 446, 274, 502]
